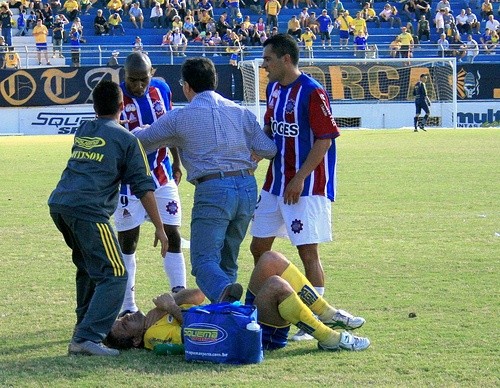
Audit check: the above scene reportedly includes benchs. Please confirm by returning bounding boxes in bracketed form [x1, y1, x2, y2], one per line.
[0, 0, 500, 62]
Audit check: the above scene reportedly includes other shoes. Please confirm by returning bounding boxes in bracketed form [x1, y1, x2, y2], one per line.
[217, 283, 243, 304]
[339, 47, 342, 49]
[420, 126, 426, 131]
[390, 26, 393, 29]
[414, 129, 418, 132]
[47, 62, 52, 64]
[346, 48, 350, 50]
[213, 54, 218, 57]
[38, 63, 41, 65]
[171, 285, 185, 293]
[59, 55, 63, 58]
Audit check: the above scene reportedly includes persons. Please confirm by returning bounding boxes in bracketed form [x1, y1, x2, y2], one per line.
[250, 34, 341, 340]
[105, 251, 370, 352]
[413, 74, 431, 132]
[114, 53, 186, 318]
[0, 0, 500, 69]
[131, 57, 278, 303]
[47, 81, 169, 357]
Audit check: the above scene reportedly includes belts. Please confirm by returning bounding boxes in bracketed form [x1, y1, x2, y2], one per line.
[198, 168, 256, 183]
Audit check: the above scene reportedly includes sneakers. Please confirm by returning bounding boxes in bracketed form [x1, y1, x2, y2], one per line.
[318, 308, 365, 330]
[291, 328, 315, 341]
[317, 331, 371, 352]
[67, 336, 120, 356]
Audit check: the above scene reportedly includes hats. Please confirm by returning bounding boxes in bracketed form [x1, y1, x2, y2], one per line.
[322, 9, 327, 13]
[115, 13, 118, 18]
[172, 16, 180, 21]
[401, 27, 407, 30]
[271, 27, 277, 30]
[136, 36, 141, 39]
[112, 50, 119, 55]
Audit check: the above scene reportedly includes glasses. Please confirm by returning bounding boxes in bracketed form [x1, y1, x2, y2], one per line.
[178, 79, 184, 86]
